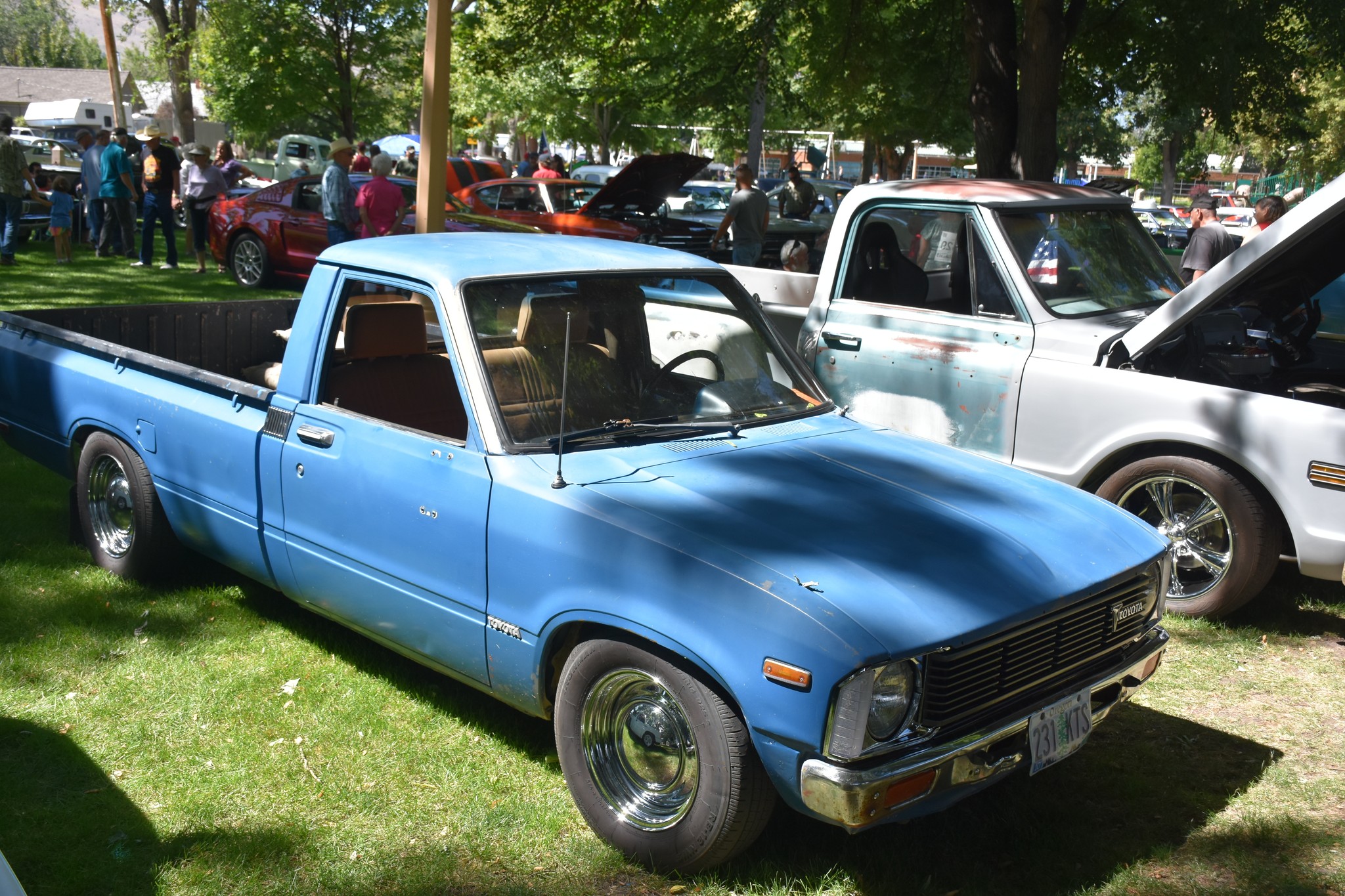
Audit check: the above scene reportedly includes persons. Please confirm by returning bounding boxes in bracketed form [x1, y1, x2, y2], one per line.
[708, 157, 976, 188]
[1239, 195, 1290, 249]
[913, 210, 964, 299]
[355, 152, 404, 295]
[392, 146, 418, 179]
[353, 142, 371, 172]
[31, 176, 74, 265]
[289, 161, 309, 179]
[0, 116, 39, 267]
[1171, 193, 1235, 284]
[178, 145, 227, 273]
[130, 123, 180, 270]
[776, 236, 809, 273]
[321, 137, 359, 246]
[369, 145, 380, 178]
[498, 151, 513, 196]
[778, 167, 817, 221]
[711, 163, 769, 267]
[24, 112, 253, 273]
[517, 151, 570, 211]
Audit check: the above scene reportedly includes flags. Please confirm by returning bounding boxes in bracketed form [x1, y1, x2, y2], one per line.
[539, 132, 550, 159]
[1027, 214, 1059, 287]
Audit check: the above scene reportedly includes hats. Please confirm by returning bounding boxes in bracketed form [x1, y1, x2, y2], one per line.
[73, 129, 90, 142]
[328, 137, 354, 155]
[1182, 195, 1219, 214]
[406, 146, 417, 154]
[524, 153, 529, 159]
[135, 125, 168, 141]
[536, 153, 554, 165]
[111, 127, 127, 136]
[736, 164, 750, 172]
[357, 142, 366, 150]
[188, 144, 210, 155]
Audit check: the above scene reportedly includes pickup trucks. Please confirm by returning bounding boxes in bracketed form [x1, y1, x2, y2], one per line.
[232, 135, 337, 187]
[1, 233, 1175, 892]
[511, 172, 1345, 619]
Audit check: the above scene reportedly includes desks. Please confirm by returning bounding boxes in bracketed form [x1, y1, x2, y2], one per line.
[20, 214, 51, 242]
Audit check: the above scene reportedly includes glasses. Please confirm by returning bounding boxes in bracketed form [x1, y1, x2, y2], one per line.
[347, 149, 356, 157]
[1252, 208, 1261, 215]
[788, 240, 800, 261]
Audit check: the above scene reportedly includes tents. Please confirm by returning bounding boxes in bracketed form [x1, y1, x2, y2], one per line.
[367, 132, 420, 160]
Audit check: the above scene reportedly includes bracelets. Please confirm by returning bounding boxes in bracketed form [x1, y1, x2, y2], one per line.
[713, 237, 718, 243]
[172, 194, 178, 198]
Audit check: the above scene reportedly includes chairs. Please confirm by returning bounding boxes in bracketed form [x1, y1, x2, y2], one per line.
[950, 218, 971, 299]
[325, 292, 623, 440]
[852, 222, 930, 307]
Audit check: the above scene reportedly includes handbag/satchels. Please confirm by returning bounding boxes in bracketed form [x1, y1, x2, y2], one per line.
[183, 195, 196, 210]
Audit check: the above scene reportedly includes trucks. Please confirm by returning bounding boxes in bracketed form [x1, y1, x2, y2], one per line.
[23, 99, 226, 160]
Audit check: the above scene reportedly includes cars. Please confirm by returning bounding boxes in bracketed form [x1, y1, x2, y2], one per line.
[423, 150, 721, 261]
[560, 151, 926, 273]
[1131, 191, 1274, 282]
[208, 173, 549, 309]
[9, 135, 83, 169]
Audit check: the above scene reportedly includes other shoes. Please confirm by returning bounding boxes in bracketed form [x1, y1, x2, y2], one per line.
[160, 263, 178, 270]
[191, 268, 206, 274]
[218, 263, 227, 274]
[130, 261, 152, 268]
[0, 255, 19, 267]
